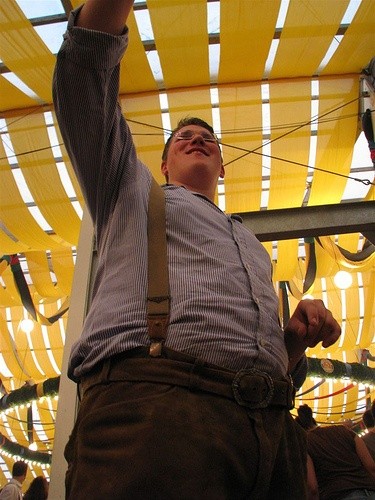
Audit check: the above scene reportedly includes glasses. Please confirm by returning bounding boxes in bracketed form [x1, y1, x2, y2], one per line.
[173, 133, 219, 142]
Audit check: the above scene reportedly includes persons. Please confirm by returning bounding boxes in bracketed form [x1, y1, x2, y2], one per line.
[295, 400, 375, 500]
[0, 461, 49, 500]
[52, 1, 341, 500]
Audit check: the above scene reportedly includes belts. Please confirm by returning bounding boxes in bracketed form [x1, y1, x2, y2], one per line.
[78, 358, 294, 410]
